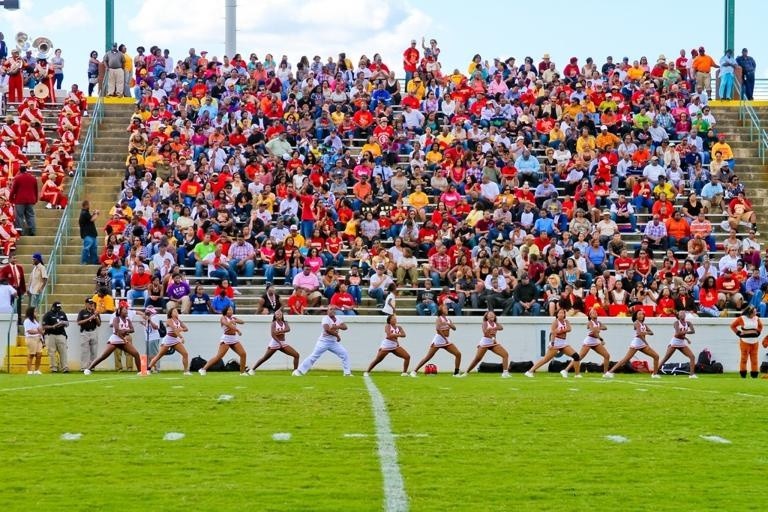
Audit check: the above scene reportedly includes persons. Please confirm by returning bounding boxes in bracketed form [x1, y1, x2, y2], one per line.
[247, 309, 299, 375]
[44, 302, 72, 373]
[85, 307, 142, 377]
[660, 311, 700, 378]
[198, 305, 250, 376]
[462, 311, 513, 379]
[524, 309, 584, 378]
[108, 301, 145, 372]
[25, 308, 44, 375]
[560, 308, 616, 379]
[147, 309, 192, 377]
[609, 309, 661, 380]
[77, 299, 98, 372]
[292, 304, 358, 376]
[762, 335, 768, 380]
[730, 306, 763, 380]
[140, 305, 164, 374]
[410, 303, 462, 377]
[362, 315, 410, 376]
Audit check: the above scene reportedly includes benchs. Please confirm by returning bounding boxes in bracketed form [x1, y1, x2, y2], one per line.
[0, 96, 73, 272]
[111, 100, 768, 317]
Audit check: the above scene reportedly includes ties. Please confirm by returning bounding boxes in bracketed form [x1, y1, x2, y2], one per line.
[13, 265, 18, 287]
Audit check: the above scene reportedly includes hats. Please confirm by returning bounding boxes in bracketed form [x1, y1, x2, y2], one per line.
[147, 305, 158, 313]
[32, 254, 42, 261]
[0, 42, 120, 222]
[85, 298, 94, 304]
[290, 225, 298, 230]
[484, 46, 729, 167]
[642, 238, 650, 244]
[228, 82, 235, 87]
[53, 302, 63, 309]
[212, 173, 219, 177]
[200, 50, 209, 54]
[724, 267, 733, 274]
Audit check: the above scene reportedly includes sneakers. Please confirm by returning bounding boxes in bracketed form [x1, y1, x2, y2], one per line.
[137, 370, 151, 376]
[574, 375, 582, 378]
[343, 373, 353, 377]
[240, 369, 255, 377]
[26, 367, 94, 376]
[560, 370, 568, 378]
[292, 370, 302, 377]
[524, 371, 535, 377]
[502, 373, 512, 377]
[453, 372, 470, 377]
[689, 374, 698, 379]
[362, 369, 419, 378]
[602, 371, 615, 378]
[199, 369, 207, 376]
[651, 373, 661, 378]
[184, 372, 193, 376]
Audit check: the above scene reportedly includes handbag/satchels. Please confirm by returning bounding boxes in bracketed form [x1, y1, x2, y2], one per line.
[548, 360, 650, 374]
[424, 363, 439, 375]
[189, 355, 243, 371]
[660, 347, 724, 375]
[478, 361, 535, 373]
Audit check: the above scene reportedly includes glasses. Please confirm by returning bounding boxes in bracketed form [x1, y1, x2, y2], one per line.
[734, 178, 739, 181]
